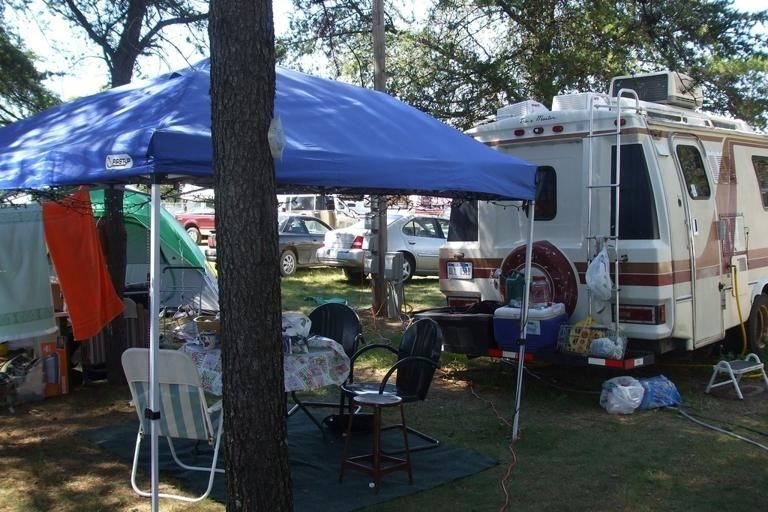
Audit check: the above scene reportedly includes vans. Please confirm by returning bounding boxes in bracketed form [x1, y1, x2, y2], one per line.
[408, 69, 768, 375]
[277, 192, 362, 231]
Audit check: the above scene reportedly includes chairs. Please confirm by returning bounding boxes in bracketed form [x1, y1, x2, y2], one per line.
[342, 318, 440, 454]
[120, 348, 223, 500]
[287, 302, 365, 440]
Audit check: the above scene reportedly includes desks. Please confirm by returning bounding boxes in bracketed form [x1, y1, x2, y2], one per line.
[55, 310, 70, 339]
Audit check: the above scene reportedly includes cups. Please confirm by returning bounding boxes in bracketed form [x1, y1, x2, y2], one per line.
[198, 327, 217, 351]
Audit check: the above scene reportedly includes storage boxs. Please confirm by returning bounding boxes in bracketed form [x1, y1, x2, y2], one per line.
[51, 275, 64, 312]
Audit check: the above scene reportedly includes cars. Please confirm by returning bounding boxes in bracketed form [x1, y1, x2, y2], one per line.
[203, 215, 335, 278]
[313, 210, 451, 285]
[174, 209, 217, 246]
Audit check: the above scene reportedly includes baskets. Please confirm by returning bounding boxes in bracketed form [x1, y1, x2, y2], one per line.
[557, 324, 628, 361]
[194, 315, 222, 336]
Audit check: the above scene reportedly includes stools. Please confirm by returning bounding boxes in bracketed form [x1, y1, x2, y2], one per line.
[704, 352, 768, 399]
[341, 393, 414, 492]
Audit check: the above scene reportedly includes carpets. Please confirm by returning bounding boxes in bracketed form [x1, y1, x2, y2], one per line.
[82, 393, 499, 512]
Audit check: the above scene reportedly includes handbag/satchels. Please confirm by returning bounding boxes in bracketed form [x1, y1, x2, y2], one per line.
[467, 300, 504, 313]
[323, 413, 375, 433]
[505, 276, 524, 307]
[637, 376, 681, 410]
